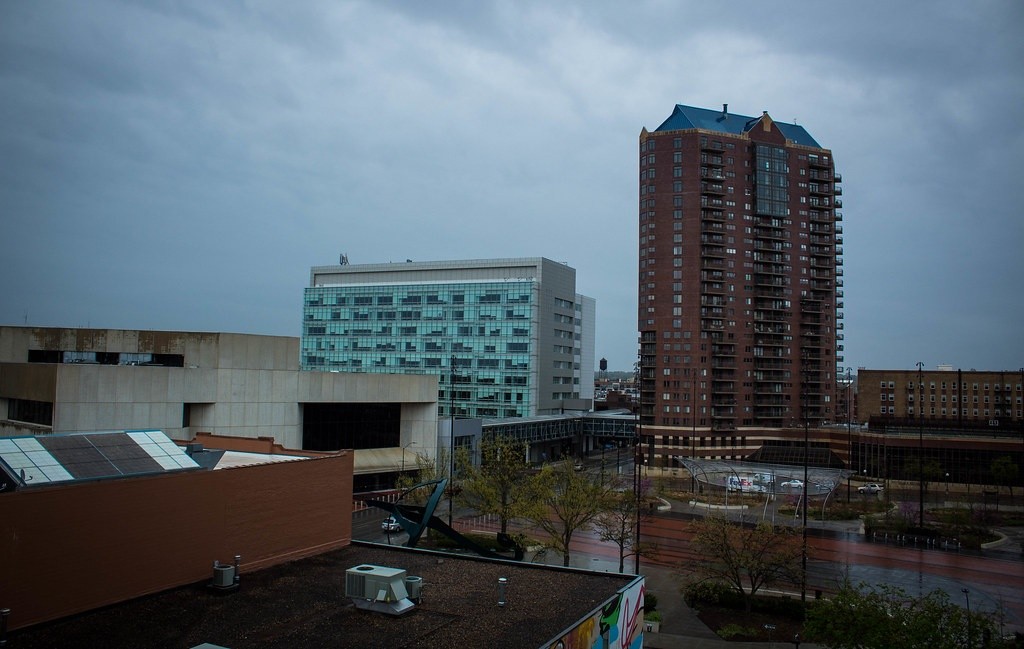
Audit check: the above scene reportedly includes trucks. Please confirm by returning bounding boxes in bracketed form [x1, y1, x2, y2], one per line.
[755, 472, 775, 486]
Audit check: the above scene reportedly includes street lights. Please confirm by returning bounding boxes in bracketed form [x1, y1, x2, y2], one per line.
[402, 441, 417, 477]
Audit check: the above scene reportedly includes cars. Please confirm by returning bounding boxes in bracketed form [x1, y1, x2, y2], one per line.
[858, 484, 884, 495]
[381, 516, 404, 533]
[781, 480, 805, 489]
[815, 481, 835, 491]
[574, 463, 586, 471]
